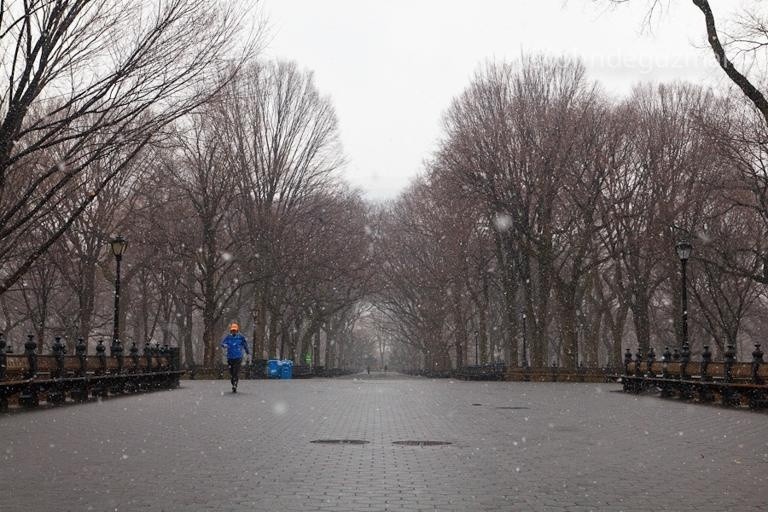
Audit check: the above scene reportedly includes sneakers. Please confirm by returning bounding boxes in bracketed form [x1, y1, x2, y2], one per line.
[232, 386, 237, 393]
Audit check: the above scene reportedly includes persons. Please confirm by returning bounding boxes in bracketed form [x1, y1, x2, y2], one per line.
[220, 324, 250, 392]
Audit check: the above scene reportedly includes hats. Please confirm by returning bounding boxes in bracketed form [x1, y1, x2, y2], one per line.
[230, 324, 239, 333]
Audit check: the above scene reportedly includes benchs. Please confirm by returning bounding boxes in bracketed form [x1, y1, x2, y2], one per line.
[0, 333, 189, 410]
[613, 344, 767, 410]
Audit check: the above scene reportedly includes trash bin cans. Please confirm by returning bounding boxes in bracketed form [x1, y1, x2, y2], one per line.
[266, 359, 293, 379]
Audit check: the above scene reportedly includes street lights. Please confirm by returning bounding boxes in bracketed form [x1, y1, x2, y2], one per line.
[475, 330, 479, 365]
[675, 239, 693, 346]
[250, 308, 260, 360]
[110, 234, 128, 340]
[522, 314, 528, 365]
